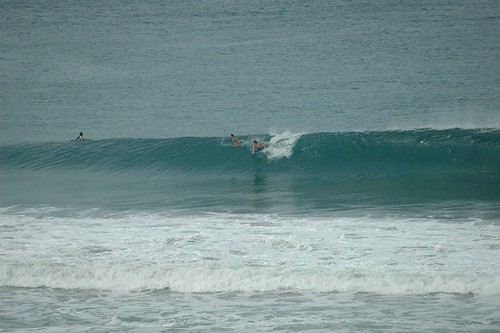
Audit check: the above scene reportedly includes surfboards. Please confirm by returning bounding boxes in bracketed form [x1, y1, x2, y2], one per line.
[255, 148, 261, 152]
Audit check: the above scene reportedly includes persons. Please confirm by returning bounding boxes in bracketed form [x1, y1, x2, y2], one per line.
[79, 132, 84, 140]
[230, 134, 238, 145]
[252, 141, 264, 150]
